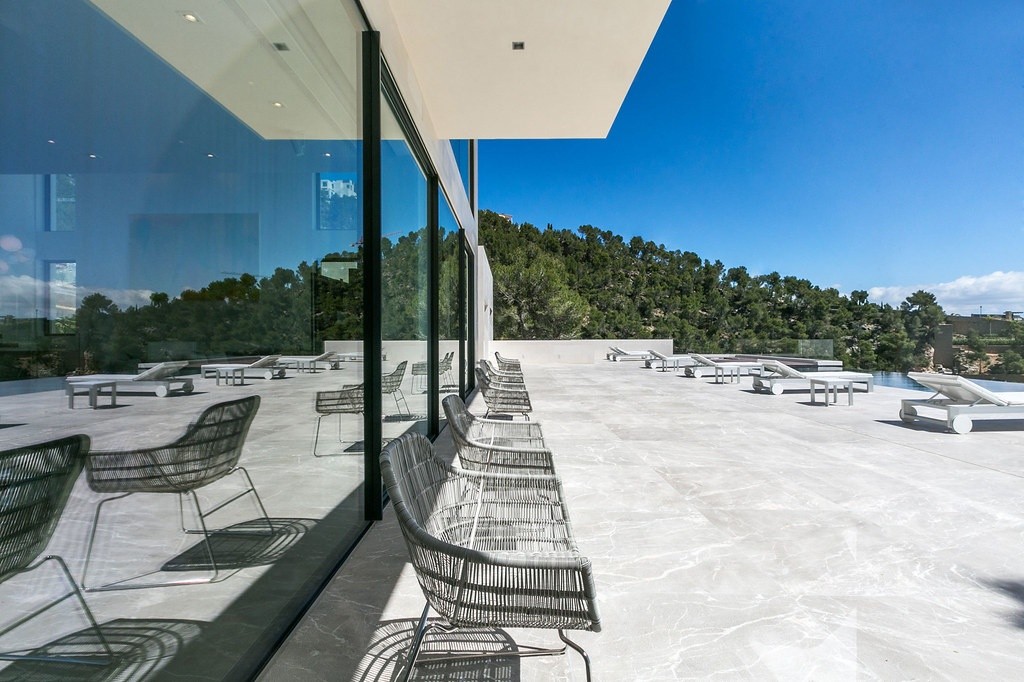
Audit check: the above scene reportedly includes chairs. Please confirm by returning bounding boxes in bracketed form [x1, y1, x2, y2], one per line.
[261, 350, 345, 374]
[484, 351, 524, 383]
[684, 353, 760, 379]
[0, 433, 120, 667]
[314, 383, 364, 457]
[338, 346, 387, 361]
[411, 352, 455, 396]
[442, 394, 558, 523]
[752, 359, 874, 396]
[645, 349, 700, 369]
[382, 360, 411, 422]
[65, 361, 193, 397]
[475, 368, 533, 445]
[899, 371, 1024, 435]
[80, 395, 275, 591]
[202, 355, 286, 380]
[378, 433, 601, 682]
[607, 346, 651, 362]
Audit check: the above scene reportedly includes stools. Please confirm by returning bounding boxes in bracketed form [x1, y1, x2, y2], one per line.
[661, 358, 680, 372]
[810, 378, 853, 407]
[715, 366, 741, 385]
[68, 381, 117, 409]
[296, 359, 317, 374]
[216, 368, 244, 386]
